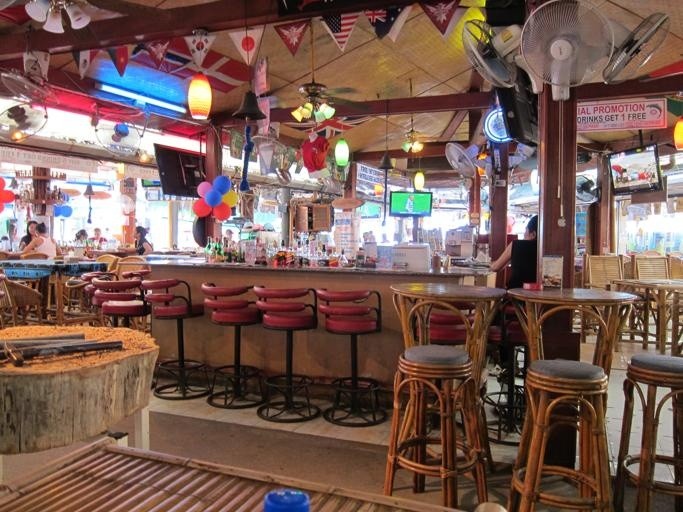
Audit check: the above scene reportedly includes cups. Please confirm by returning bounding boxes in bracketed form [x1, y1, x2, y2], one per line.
[263, 489, 310, 511]
[430, 255, 441, 271]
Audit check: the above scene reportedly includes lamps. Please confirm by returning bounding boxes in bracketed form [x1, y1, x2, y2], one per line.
[412, 156, 427, 192]
[672, 113, 683, 150]
[93, 82, 187, 118]
[334, 115, 348, 173]
[402, 77, 426, 154]
[184, 31, 213, 124]
[22, 1, 90, 38]
[288, 81, 337, 125]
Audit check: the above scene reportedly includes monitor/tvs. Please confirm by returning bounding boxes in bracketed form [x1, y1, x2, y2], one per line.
[486, 0, 539, 147]
[607, 143, 664, 196]
[355, 201, 381, 218]
[389, 191, 432, 217]
[153, 142, 207, 198]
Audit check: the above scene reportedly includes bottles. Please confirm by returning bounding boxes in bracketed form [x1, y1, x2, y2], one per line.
[101, 238, 121, 250]
[18, 182, 63, 200]
[203, 235, 348, 267]
[14, 169, 67, 181]
[56, 239, 99, 251]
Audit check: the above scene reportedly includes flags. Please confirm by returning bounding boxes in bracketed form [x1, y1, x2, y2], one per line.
[283, 106, 375, 141]
[128, 21, 262, 94]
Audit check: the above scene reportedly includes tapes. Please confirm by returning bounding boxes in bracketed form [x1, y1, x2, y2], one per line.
[524, 283, 543, 290]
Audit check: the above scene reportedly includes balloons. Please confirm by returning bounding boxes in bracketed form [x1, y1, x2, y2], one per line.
[0, 176, 15, 212]
[191, 175, 239, 221]
[53, 192, 72, 218]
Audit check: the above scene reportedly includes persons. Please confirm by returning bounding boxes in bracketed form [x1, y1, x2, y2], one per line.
[611, 164, 655, 183]
[485, 214, 536, 377]
[18, 220, 38, 250]
[404, 195, 415, 213]
[300, 130, 331, 181]
[134, 226, 152, 255]
[72, 229, 88, 242]
[224, 229, 235, 245]
[88, 227, 107, 242]
[13, 222, 62, 260]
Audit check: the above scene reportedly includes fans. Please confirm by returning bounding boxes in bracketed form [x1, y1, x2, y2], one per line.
[573, 173, 601, 204]
[442, 138, 492, 181]
[250, 121, 288, 157]
[0, 48, 53, 106]
[0, 102, 49, 146]
[517, 2, 619, 104]
[267, 18, 373, 112]
[459, 14, 547, 95]
[506, 137, 541, 172]
[589, 10, 671, 86]
[90, 108, 146, 159]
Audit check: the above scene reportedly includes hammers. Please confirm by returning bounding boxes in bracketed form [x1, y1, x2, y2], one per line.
[6, 341, 122, 366]
